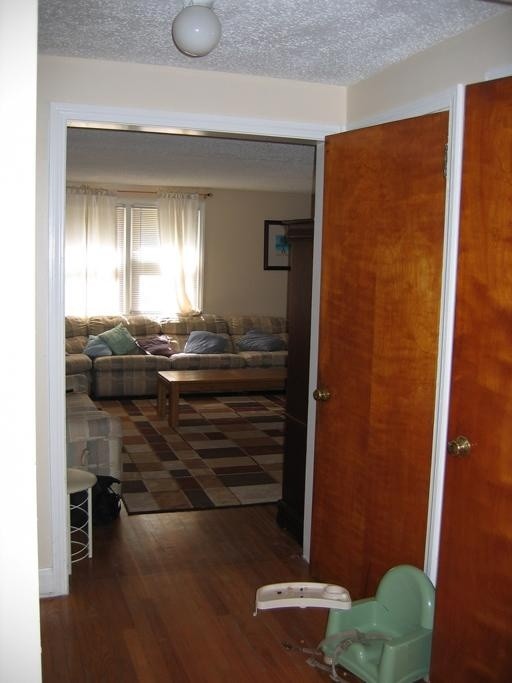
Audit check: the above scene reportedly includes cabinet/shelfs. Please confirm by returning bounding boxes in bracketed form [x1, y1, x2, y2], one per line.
[277, 218, 314, 541]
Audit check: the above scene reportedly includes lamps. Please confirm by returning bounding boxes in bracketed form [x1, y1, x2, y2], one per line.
[172, 2, 223, 60]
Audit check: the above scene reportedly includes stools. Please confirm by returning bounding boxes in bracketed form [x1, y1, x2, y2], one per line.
[67, 466, 98, 576]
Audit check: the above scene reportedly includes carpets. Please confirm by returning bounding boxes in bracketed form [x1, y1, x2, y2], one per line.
[93, 393, 288, 514]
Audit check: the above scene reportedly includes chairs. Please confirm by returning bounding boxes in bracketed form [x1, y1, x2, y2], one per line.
[320, 566, 436, 683]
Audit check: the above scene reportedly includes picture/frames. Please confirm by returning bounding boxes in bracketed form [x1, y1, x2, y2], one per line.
[264, 219, 289, 271]
